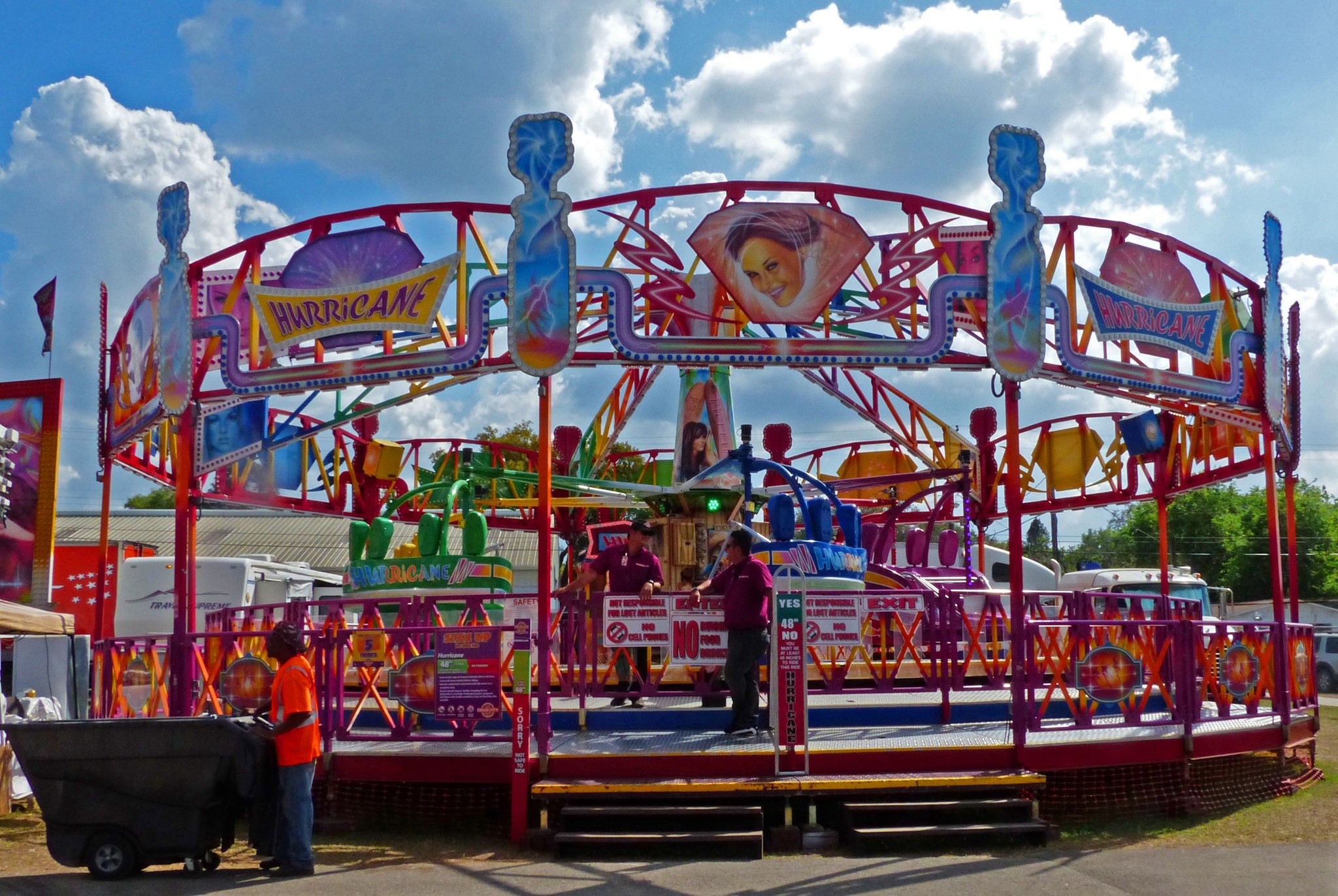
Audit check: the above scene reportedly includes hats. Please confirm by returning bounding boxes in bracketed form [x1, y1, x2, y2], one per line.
[273, 621, 306, 650]
[631, 520, 655, 533]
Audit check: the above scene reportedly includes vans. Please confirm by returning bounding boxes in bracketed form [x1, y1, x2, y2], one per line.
[1310, 631, 1338, 693]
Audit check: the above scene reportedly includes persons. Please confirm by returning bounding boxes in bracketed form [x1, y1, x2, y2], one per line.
[239, 621, 322, 876]
[552, 519, 665, 707]
[688, 531, 772, 736]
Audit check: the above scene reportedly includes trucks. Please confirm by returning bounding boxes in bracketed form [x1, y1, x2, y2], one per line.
[1053, 566, 1239, 680]
[114, 549, 369, 638]
[869, 541, 1061, 625]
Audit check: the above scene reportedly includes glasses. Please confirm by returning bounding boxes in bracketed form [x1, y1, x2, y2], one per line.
[726, 543, 739, 549]
[635, 531, 652, 537]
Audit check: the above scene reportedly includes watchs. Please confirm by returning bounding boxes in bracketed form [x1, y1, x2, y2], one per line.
[690, 587, 700, 590]
[647, 580, 654, 587]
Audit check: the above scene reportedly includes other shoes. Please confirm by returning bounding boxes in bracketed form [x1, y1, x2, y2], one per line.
[260, 856, 287, 869]
[631, 699, 644, 708]
[611, 698, 626, 706]
[724, 723, 757, 736]
[272, 863, 314, 877]
